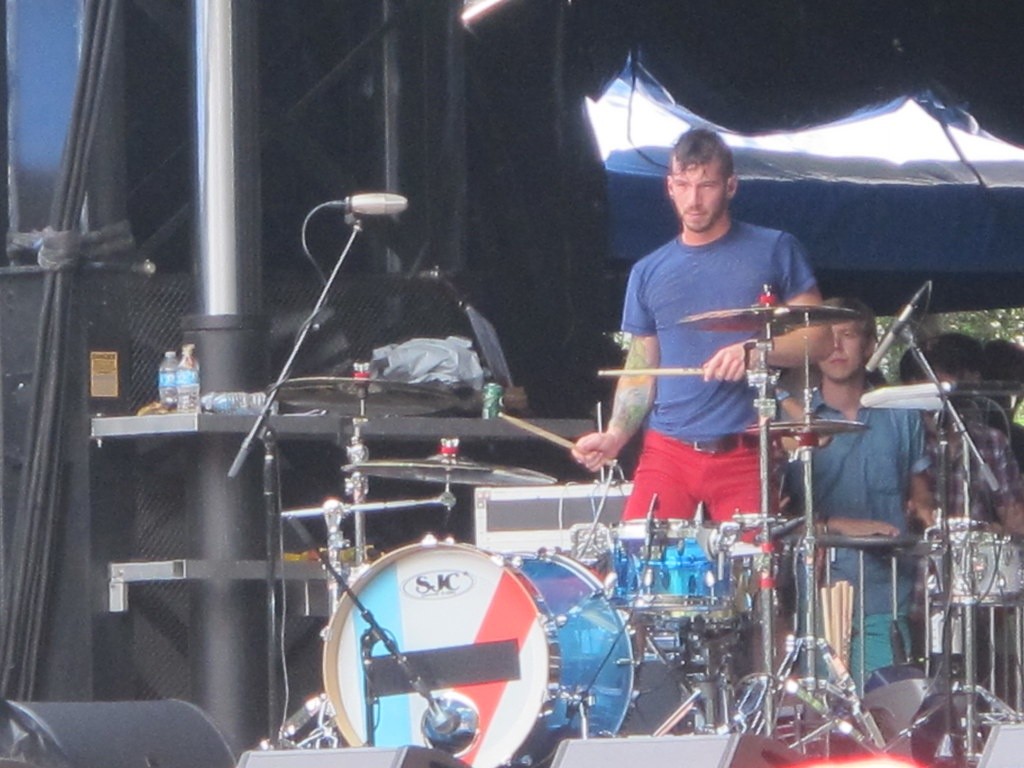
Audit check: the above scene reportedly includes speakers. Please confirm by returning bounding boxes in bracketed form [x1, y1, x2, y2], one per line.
[550, 734, 806, 768]
[0, 260, 467, 759]
[975, 725, 1024, 768]
[235, 744, 466, 768]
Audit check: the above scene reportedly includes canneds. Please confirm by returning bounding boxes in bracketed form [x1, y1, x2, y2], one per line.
[480, 382, 505, 419]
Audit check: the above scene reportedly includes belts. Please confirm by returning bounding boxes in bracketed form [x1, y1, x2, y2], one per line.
[686, 432, 772, 453]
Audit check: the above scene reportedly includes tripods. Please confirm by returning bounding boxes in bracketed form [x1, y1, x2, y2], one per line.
[650, 311, 1024, 768]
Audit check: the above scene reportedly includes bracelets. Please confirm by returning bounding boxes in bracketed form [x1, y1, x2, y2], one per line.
[777, 388, 793, 404]
[824, 517, 829, 535]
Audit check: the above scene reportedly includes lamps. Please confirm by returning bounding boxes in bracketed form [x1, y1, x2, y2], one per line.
[458, 0, 533, 41]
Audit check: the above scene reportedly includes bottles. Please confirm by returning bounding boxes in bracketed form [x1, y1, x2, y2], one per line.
[177, 351, 201, 412]
[158, 351, 178, 405]
[201, 392, 279, 416]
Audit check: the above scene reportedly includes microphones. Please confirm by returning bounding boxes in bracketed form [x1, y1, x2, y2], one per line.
[755, 516, 804, 544]
[865, 288, 929, 373]
[430, 707, 459, 735]
[325, 193, 408, 214]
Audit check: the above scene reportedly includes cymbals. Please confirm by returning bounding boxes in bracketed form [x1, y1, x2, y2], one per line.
[340, 454, 559, 489]
[745, 417, 873, 435]
[674, 302, 865, 332]
[856, 374, 1024, 413]
[268, 374, 462, 416]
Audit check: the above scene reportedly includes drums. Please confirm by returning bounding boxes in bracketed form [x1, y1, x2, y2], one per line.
[731, 512, 828, 618]
[928, 529, 1024, 610]
[605, 516, 737, 625]
[320, 537, 638, 768]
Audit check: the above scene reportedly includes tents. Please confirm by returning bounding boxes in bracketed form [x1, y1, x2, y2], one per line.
[581, 52, 1024, 336]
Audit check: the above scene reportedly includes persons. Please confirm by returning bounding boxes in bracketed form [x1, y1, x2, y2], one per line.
[569, 128, 835, 675]
[774, 383, 832, 516]
[898, 336, 1024, 677]
[777, 293, 943, 699]
